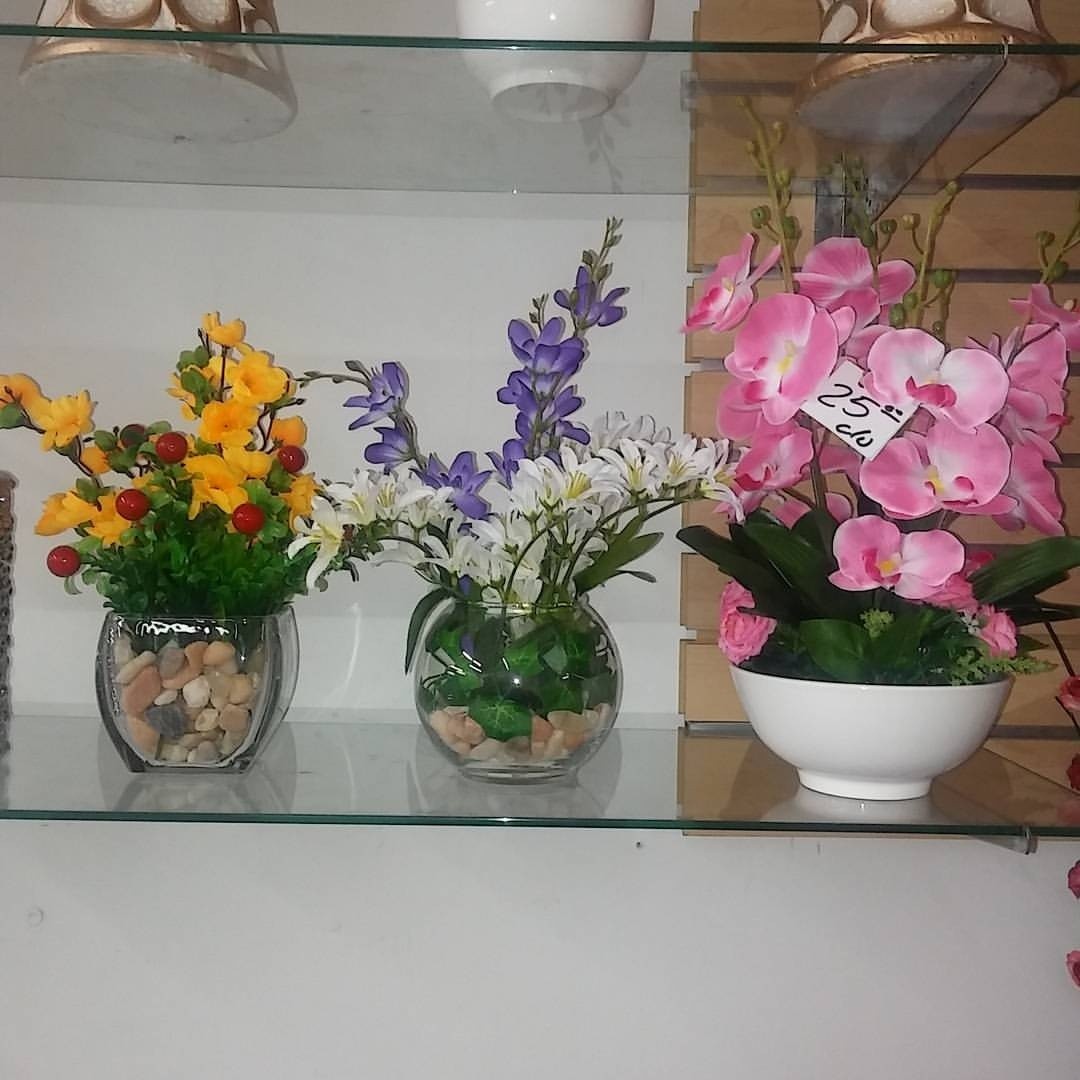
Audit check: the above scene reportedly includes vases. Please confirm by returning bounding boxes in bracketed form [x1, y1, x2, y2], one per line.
[728, 660, 1017, 801]
[798, 0, 1066, 145]
[16, 0, 300, 145]
[94, 607, 300, 776]
[454, 0, 654, 124]
[413, 599, 624, 786]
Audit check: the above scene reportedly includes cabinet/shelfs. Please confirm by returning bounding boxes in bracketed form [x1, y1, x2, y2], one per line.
[0, 19, 1080, 856]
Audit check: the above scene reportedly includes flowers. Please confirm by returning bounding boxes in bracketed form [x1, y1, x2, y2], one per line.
[0, 182, 1080, 740]
